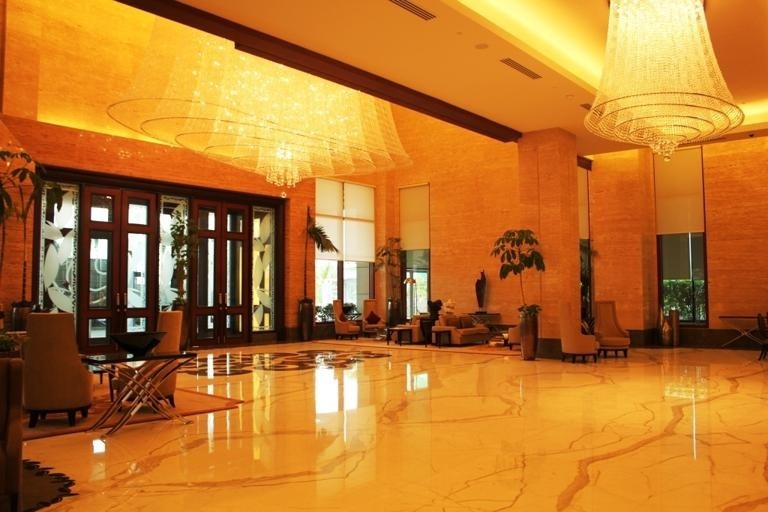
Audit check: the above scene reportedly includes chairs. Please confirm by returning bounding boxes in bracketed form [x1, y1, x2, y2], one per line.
[593, 300, 631, 358]
[558, 300, 599, 364]
[21, 313, 94, 428]
[363, 299, 385, 338]
[757, 314, 768, 361]
[332, 300, 360, 341]
[507, 325, 521, 351]
[108, 311, 185, 412]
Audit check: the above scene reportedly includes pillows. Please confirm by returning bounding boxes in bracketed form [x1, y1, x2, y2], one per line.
[412, 315, 430, 325]
[457, 314, 474, 328]
[366, 310, 381, 324]
[438, 315, 459, 329]
[338, 312, 347, 322]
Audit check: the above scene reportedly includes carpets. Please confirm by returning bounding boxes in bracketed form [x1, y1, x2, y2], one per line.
[22, 380, 245, 443]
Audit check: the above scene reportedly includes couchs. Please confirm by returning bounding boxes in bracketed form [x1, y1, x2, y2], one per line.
[396, 320, 423, 343]
[432, 321, 489, 347]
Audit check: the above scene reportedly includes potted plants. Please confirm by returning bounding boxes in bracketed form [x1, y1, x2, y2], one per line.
[168, 209, 194, 349]
[298, 207, 339, 343]
[0, 151, 52, 332]
[490, 228, 547, 361]
[374, 238, 407, 326]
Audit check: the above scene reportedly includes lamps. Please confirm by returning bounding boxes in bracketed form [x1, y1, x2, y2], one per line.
[105, 14, 414, 187]
[585, 0, 745, 162]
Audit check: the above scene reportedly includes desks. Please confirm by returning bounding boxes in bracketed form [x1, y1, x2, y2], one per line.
[718, 315, 768, 349]
[388, 328, 414, 347]
[425, 330, 452, 349]
[84, 351, 197, 435]
